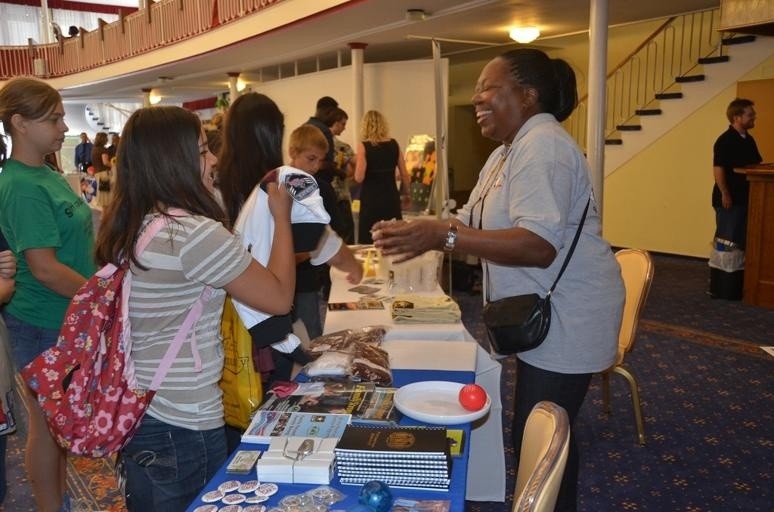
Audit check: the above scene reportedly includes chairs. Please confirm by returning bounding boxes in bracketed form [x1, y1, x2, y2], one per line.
[601, 248, 657, 443]
[510, 399, 574, 510]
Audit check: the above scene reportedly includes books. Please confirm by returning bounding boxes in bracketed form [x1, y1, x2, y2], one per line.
[242, 379, 453, 494]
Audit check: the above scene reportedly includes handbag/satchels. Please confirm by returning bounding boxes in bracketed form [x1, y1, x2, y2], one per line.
[483, 294, 551, 356]
[219, 293, 263, 429]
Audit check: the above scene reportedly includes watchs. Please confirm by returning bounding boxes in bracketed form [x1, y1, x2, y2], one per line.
[444, 218, 459, 255]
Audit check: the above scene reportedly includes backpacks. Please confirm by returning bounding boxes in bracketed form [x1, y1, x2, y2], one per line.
[21, 207, 211, 459]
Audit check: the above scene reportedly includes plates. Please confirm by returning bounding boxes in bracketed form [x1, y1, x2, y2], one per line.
[392, 379, 492, 425]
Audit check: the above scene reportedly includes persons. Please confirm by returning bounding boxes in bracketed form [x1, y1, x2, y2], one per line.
[2, 95, 412, 243]
[707, 97, 763, 300]
[214, 91, 366, 402]
[369, 47, 624, 511]
[53, 24, 80, 41]
[0, 230, 17, 507]
[290, 123, 348, 338]
[92, 105, 297, 512]
[0, 74, 97, 512]
